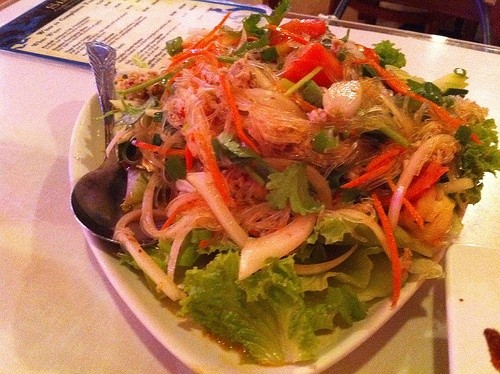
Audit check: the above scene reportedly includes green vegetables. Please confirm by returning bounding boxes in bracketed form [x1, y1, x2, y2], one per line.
[104, 4, 498, 366]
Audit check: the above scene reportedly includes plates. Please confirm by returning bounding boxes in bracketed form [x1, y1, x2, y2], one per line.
[440, 238, 500, 373]
[72, 72, 470, 373]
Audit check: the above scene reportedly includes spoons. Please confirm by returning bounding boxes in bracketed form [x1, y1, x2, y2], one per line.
[70, 41, 168, 246]
